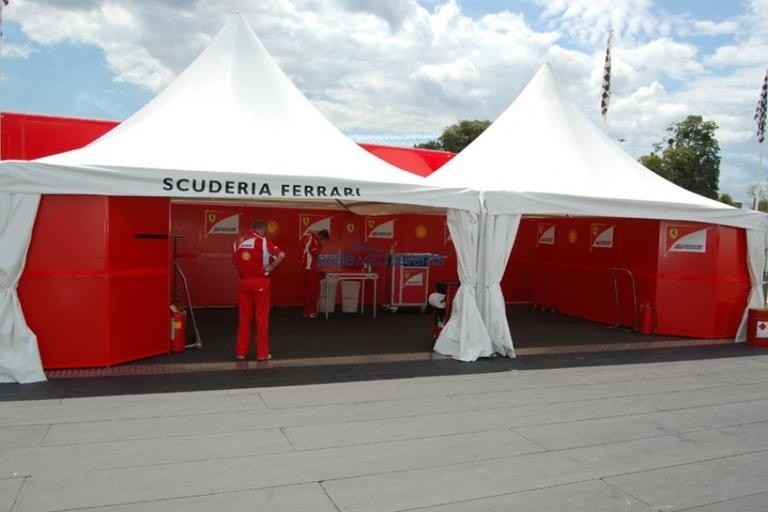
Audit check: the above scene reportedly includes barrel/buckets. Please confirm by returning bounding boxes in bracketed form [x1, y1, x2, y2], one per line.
[745, 308, 768, 346]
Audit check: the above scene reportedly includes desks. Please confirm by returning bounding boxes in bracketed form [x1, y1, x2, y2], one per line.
[325, 270, 379, 319]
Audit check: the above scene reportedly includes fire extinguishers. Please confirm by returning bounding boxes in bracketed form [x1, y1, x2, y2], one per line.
[638, 301, 653, 335]
[170, 302, 187, 353]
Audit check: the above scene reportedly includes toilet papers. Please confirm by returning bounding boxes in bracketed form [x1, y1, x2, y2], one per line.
[429, 292, 447, 308]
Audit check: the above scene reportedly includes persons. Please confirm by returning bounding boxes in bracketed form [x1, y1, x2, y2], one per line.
[301, 229, 330, 319]
[230, 219, 287, 360]
